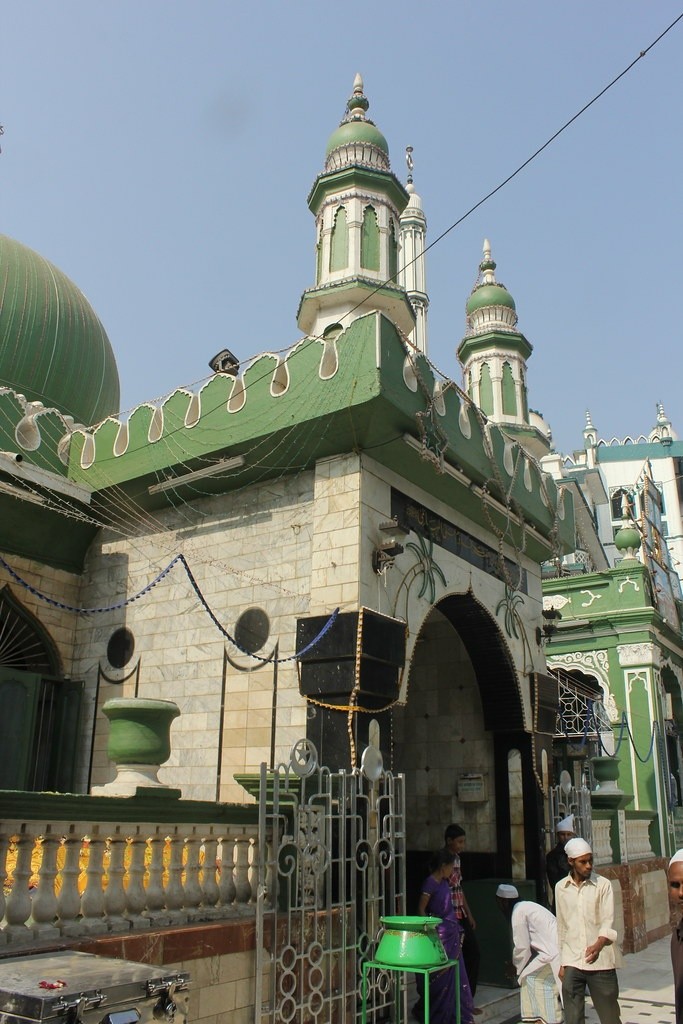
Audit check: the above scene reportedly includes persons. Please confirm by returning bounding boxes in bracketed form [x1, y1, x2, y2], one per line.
[546, 814, 574, 917]
[555, 838, 623, 1024]
[669, 848, 683, 1024]
[412, 850, 475, 1024]
[444, 824, 483, 1015]
[496, 884, 566, 1024]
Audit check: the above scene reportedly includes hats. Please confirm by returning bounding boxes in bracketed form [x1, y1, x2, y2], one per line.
[496, 884, 519, 898]
[556, 814, 574, 833]
[564, 838, 593, 859]
[669, 848, 683, 868]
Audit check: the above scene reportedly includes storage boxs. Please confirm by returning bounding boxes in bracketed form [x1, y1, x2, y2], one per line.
[0, 949, 192, 1024]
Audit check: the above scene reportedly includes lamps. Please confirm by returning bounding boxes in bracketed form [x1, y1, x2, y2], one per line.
[369, 514, 410, 578]
[208, 349, 239, 376]
[534, 605, 563, 648]
